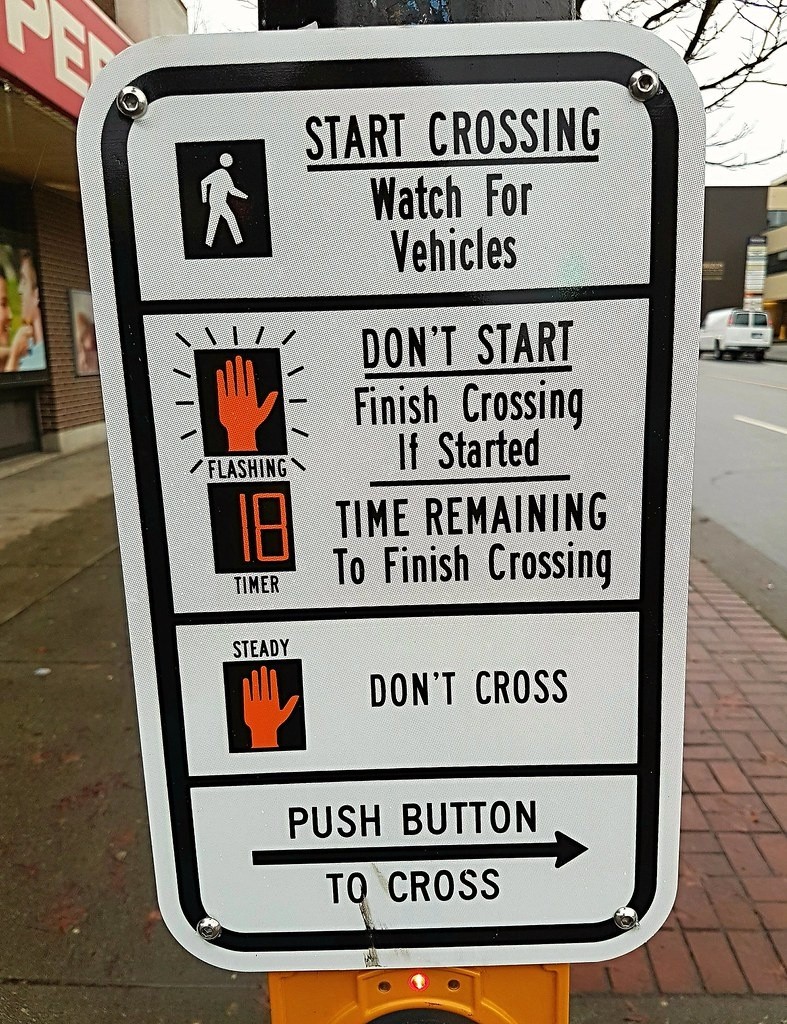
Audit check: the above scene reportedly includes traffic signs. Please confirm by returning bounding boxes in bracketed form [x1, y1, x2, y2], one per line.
[69, 11, 688, 967]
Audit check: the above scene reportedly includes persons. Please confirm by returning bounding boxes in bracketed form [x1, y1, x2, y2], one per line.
[0, 253, 47, 372]
[76, 312, 98, 372]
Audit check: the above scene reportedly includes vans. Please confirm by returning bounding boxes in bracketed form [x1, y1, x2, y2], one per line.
[696, 307, 773, 363]
